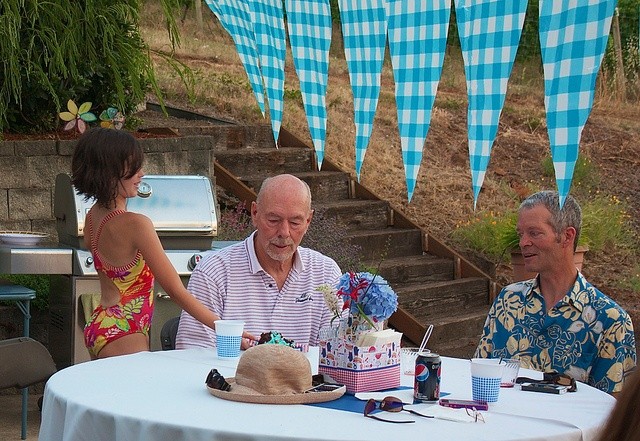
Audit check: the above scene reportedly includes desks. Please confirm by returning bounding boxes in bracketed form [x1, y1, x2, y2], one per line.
[0, 276, 37, 440]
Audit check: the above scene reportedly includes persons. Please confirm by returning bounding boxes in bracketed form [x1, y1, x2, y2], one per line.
[473, 191, 638, 402]
[174, 175, 350, 351]
[71, 127, 260, 362]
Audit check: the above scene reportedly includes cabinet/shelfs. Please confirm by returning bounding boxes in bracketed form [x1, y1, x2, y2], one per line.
[46, 274, 190, 370]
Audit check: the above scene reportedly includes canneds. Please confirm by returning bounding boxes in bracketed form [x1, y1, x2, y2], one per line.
[413, 353, 441, 403]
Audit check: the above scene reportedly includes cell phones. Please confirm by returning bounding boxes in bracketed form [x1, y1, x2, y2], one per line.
[440, 399, 488, 411]
[520, 382, 567, 395]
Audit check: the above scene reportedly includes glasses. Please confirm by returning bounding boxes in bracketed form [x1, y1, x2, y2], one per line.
[363, 396, 434, 423]
[205, 368, 231, 390]
[466, 406, 484, 422]
[513, 373, 577, 392]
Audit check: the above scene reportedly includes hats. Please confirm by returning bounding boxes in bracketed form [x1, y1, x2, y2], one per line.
[206, 343, 346, 402]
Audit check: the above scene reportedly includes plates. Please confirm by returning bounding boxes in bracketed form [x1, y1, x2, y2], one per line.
[0, 231, 50, 245]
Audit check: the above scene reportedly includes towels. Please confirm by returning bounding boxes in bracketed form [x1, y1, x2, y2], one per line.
[80, 293, 102, 324]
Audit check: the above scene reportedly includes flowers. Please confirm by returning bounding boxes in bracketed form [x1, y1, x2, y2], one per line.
[316, 269, 399, 334]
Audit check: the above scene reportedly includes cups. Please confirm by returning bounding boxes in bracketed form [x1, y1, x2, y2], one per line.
[213, 320, 245, 361]
[500, 360, 520, 388]
[401, 348, 430, 376]
[471, 360, 506, 404]
